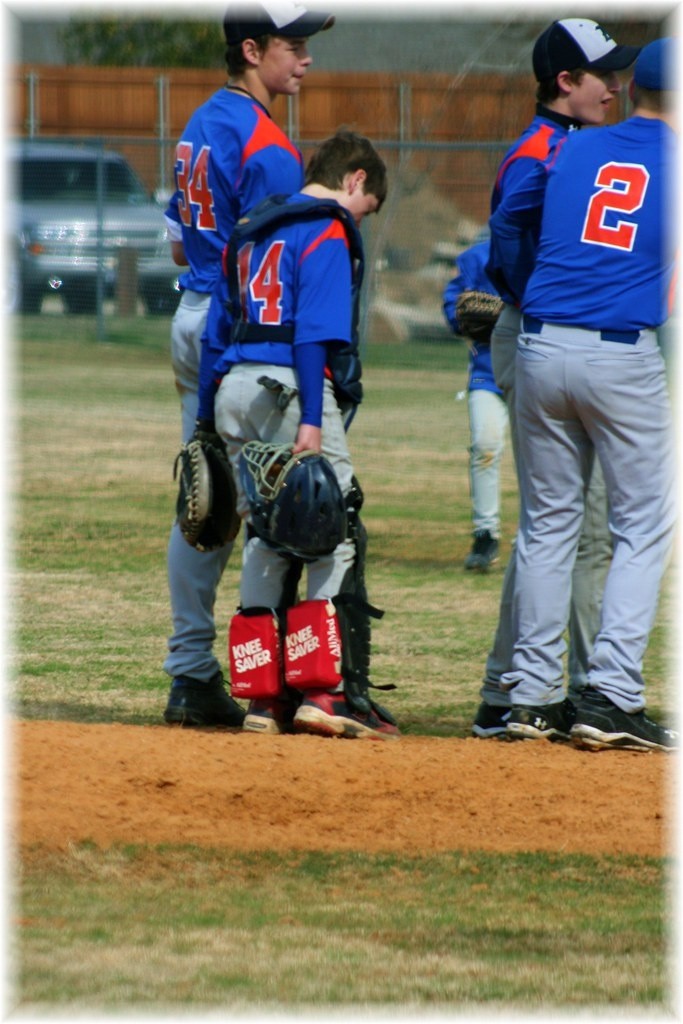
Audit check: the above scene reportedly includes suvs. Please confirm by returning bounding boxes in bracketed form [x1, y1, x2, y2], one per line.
[2, 140, 185, 317]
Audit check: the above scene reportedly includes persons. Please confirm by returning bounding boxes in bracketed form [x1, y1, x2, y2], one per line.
[472, 17, 642, 744]
[499, 35, 682, 754]
[161, 0, 336, 731]
[173, 133, 405, 740]
[443, 223, 501, 573]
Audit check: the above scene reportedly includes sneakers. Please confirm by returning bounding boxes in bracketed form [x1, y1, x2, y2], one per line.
[294, 688, 401, 740]
[243, 692, 297, 735]
[506, 698, 577, 739]
[363, 697, 396, 727]
[463, 530, 501, 571]
[570, 687, 682, 753]
[471, 702, 511, 737]
[163, 670, 247, 729]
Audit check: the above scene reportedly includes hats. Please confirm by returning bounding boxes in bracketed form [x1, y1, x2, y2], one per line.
[532, 19, 639, 83]
[222, 2, 336, 41]
[633, 36, 682, 90]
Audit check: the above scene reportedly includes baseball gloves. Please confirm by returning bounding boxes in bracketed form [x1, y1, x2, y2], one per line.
[176, 441, 241, 554]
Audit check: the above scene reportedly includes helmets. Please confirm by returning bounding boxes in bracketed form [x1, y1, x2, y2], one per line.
[238, 443, 349, 563]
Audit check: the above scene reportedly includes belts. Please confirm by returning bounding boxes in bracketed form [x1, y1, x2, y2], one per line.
[521, 318, 641, 346]
